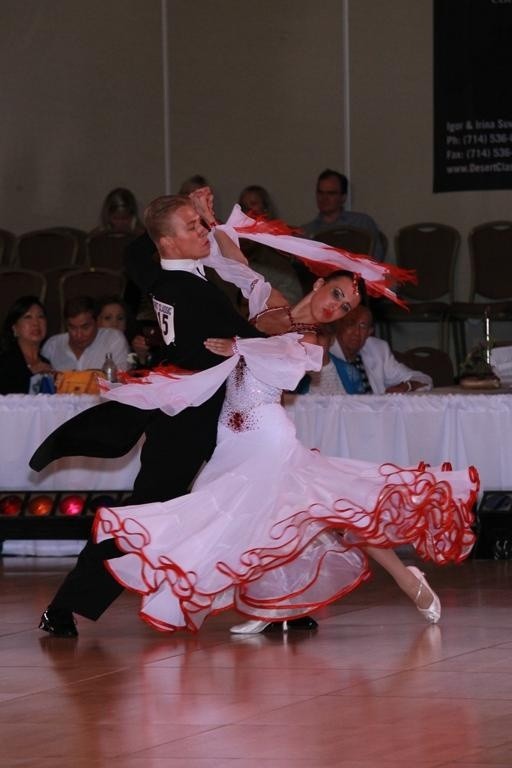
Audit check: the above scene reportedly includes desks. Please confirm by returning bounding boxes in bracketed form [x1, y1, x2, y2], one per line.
[0, 392, 512, 515]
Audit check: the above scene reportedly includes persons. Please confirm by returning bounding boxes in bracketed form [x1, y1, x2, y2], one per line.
[25, 191, 321, 642]
[1, 166, 438, 396]
[188, 183, 445, 637]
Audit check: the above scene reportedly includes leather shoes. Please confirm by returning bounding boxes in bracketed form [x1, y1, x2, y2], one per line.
[39, 608, 78, 638]
[289, 616, 320, 630]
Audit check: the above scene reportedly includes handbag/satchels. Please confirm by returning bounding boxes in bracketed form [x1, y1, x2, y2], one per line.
[56, 370, 105, 396]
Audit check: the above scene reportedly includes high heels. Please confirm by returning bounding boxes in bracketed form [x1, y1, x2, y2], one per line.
[404, 566, 441, 625]
[230, 616, 289, 634]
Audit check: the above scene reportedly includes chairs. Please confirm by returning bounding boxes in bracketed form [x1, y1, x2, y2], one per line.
[447, 219, 512, 377]
[0, 225, 138, 336]
[379, 221, 461, 362]
[306, 225, 377, 304]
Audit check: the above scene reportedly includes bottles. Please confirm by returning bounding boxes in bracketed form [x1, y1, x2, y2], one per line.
[103, 350, 117, 386]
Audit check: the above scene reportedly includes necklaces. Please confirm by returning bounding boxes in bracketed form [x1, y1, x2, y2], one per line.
[287, 304, 321, 334]
